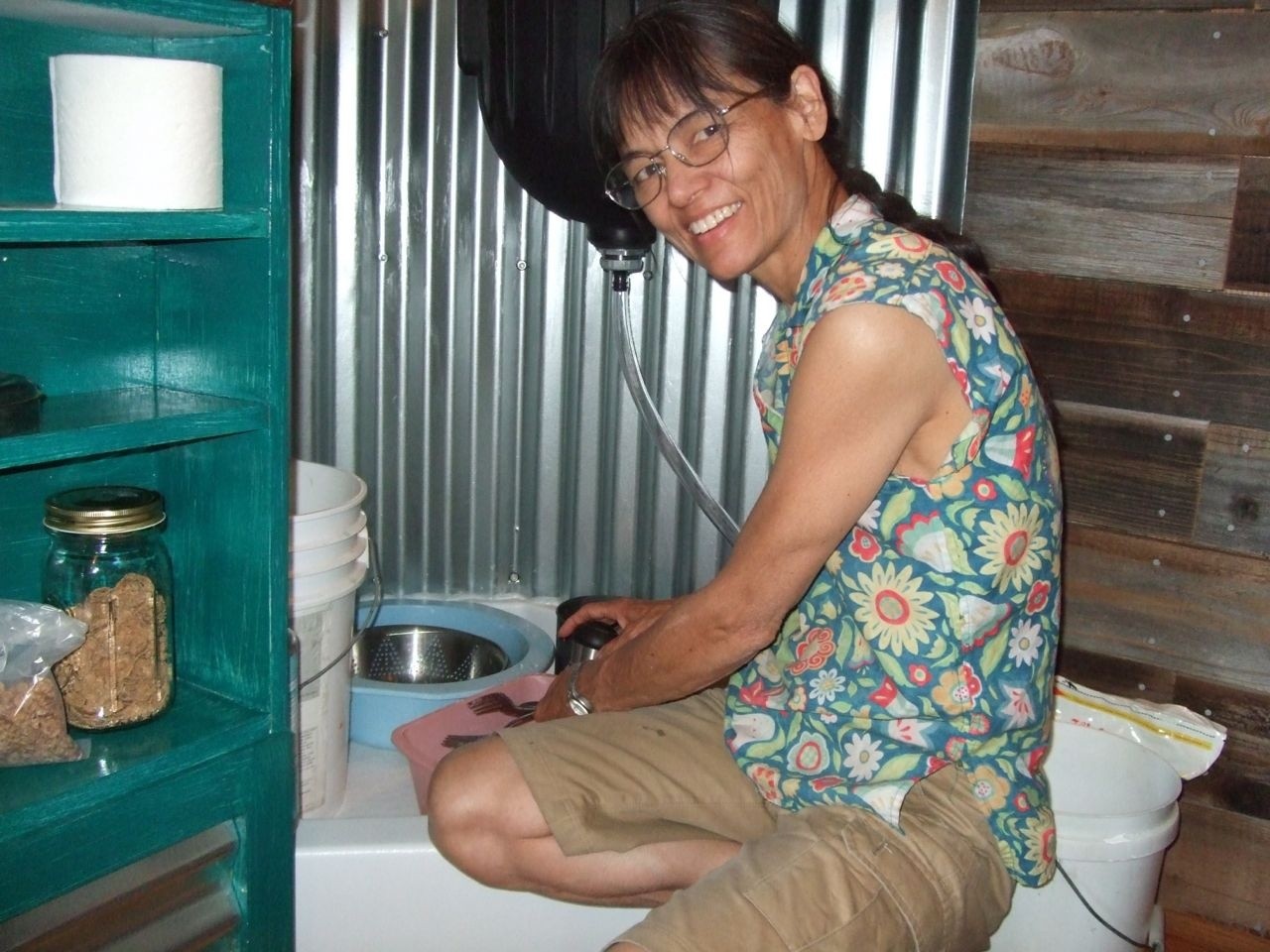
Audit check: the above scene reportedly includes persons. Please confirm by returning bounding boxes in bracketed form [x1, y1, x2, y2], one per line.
[427, 0, 1064, 952]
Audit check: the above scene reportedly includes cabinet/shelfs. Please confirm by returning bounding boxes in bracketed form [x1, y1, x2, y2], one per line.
[0, 0, 298, 952]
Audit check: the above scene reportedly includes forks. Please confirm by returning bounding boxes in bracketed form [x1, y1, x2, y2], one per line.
[439, 692, 539, 748]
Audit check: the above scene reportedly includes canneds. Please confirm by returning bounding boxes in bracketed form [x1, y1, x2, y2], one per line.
[41, 485, 174, 731]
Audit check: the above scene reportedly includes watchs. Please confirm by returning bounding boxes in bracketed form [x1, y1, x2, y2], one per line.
[567, 660, 592, 716]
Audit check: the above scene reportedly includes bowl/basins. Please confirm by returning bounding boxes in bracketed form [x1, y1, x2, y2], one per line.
[554, 594, 637, 673]
[348, 598, 553, 749]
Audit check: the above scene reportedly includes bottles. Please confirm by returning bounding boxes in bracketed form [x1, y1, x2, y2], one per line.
[42, 486, 175, 731]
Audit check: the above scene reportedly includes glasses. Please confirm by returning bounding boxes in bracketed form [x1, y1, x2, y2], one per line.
[605, 85, 776, 211]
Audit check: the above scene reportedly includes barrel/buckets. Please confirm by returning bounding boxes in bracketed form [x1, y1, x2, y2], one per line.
[285, 459, 382, 817]
[985, 719, 1181, 952]
